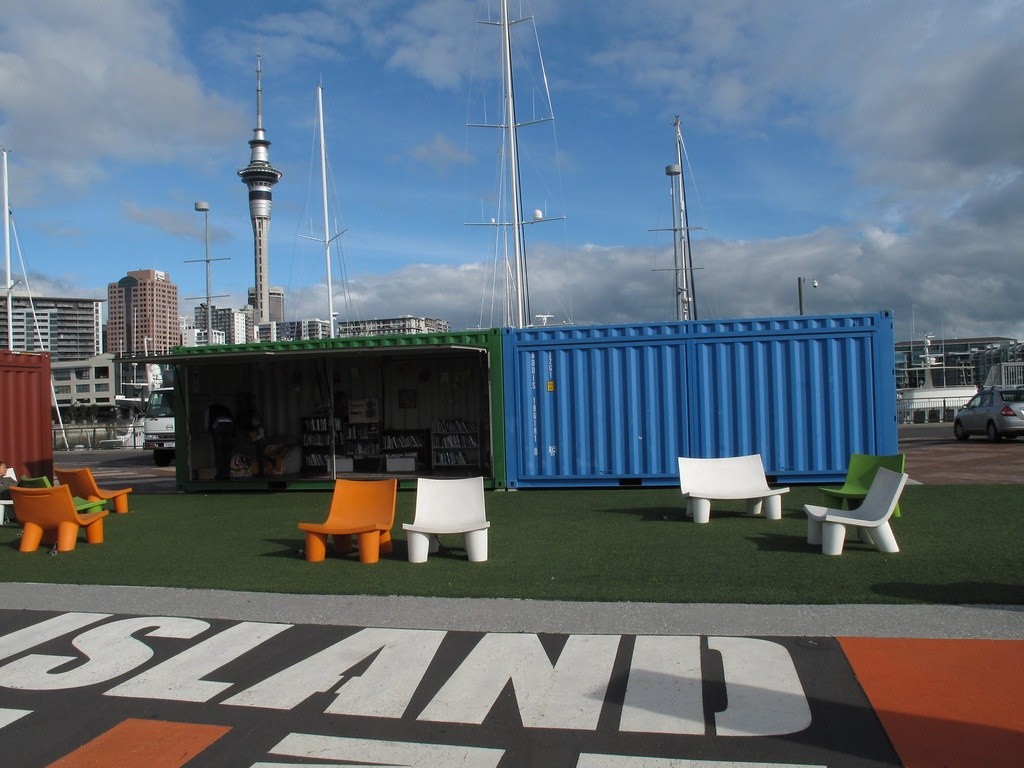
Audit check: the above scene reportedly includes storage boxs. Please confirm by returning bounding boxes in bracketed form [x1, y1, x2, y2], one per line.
[364, 455, 387, 473]
[385, 453, 418, 471]
[327, 455, 353, 473]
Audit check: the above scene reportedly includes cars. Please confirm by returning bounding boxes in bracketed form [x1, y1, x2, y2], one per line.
[953, 385, 1024, 443]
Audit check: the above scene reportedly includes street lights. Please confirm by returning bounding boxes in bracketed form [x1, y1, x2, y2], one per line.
[665, 164, 684, 321]
[194, 201, 213, 345]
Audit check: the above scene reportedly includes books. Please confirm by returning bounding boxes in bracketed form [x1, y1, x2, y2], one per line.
[303, 417, 343, 467]
[433, 416, 480, 464]
[345, 424, 380, 457]
[382, 431, 424, 449]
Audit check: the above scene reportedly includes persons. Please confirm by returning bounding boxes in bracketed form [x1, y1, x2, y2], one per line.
[250, 412, 266, 463]
[0, 462, 19, 500]
[204, 401, 236, 481]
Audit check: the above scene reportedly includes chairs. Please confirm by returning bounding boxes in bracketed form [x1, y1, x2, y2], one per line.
[0, 467, 18, 526]
[678, 454, 790, 523]
[298, 479, 397, 563]
[8, 484, 109, 552]
[819, 454, 906, 517]
[20, 474, 107, 513]
[403, 477, 490, 563]
[803, 466, 908, 556]
[54, 468, 132, 513]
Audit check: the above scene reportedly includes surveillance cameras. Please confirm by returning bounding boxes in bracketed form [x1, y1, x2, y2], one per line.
[814, 283, 818, 287]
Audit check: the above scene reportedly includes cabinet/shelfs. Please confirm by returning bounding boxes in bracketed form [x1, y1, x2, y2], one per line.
[299, 417, 481, 471]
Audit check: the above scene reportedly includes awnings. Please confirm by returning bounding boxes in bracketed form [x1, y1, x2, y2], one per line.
[111, 343, 489, 365]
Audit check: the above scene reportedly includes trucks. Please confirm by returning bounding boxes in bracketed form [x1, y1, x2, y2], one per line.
[139, 386, 176, 467]
